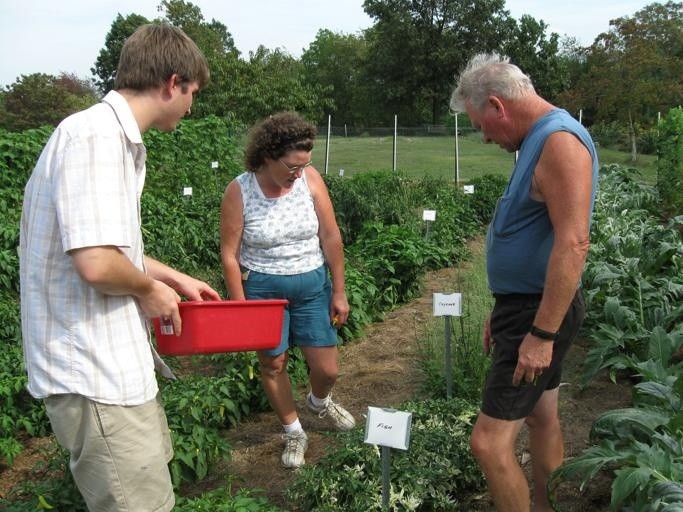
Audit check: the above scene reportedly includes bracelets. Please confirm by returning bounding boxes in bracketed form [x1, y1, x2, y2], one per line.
[530, 326, 560, 340]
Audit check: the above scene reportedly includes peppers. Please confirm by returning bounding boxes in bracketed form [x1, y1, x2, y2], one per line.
[331, 314, 339, 327]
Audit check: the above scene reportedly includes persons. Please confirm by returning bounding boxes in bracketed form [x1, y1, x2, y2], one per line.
[450, 53, 598, 512]
[20, 24, 222, 512]
[220, 111, 356, 467]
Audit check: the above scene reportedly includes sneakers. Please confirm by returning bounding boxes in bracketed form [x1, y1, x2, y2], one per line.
[305, 395, 357, 431]
[280, 423, 310, 469]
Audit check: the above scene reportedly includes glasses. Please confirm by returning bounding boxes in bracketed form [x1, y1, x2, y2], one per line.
[278, 157, 313, 175]
